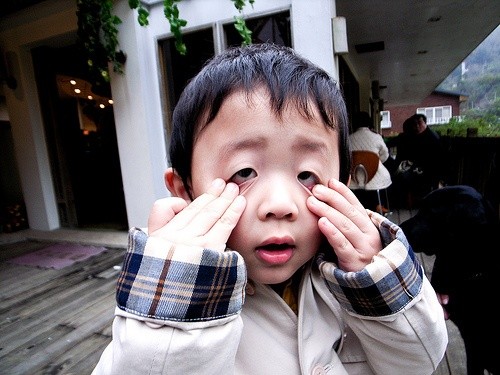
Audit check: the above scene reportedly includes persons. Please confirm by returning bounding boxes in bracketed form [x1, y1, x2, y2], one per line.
[92, 44, 450, 375]
[341, 110, 440, 215]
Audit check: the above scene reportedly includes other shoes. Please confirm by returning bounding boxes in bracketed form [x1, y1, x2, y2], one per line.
[376, 204, 393, 216]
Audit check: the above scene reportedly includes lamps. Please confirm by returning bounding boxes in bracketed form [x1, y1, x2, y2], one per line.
[333, 17, 348, 55]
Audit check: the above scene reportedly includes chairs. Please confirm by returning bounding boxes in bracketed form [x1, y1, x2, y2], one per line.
[351, 150, 390, 221]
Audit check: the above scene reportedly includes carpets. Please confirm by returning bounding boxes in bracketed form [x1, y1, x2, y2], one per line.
[13, 241, 108, 269]
